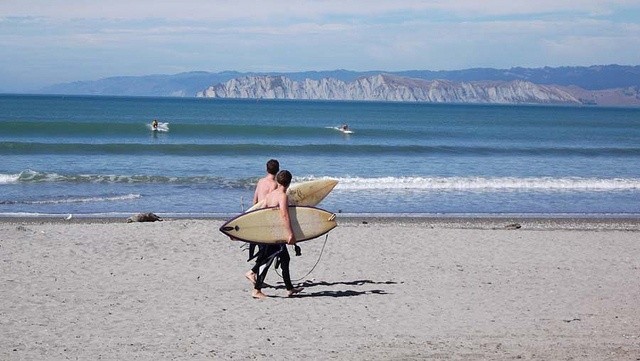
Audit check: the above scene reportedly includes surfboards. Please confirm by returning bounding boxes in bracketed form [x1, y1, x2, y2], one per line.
[219, 205, 337, 244]
[244, 180, 338, 212]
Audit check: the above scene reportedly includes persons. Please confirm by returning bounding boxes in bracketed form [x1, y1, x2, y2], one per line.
[252, 170, 304, 299]
[152, 119, 158, 128]
[341, 123, 349, 131]
[244, 159, 280, 288]
